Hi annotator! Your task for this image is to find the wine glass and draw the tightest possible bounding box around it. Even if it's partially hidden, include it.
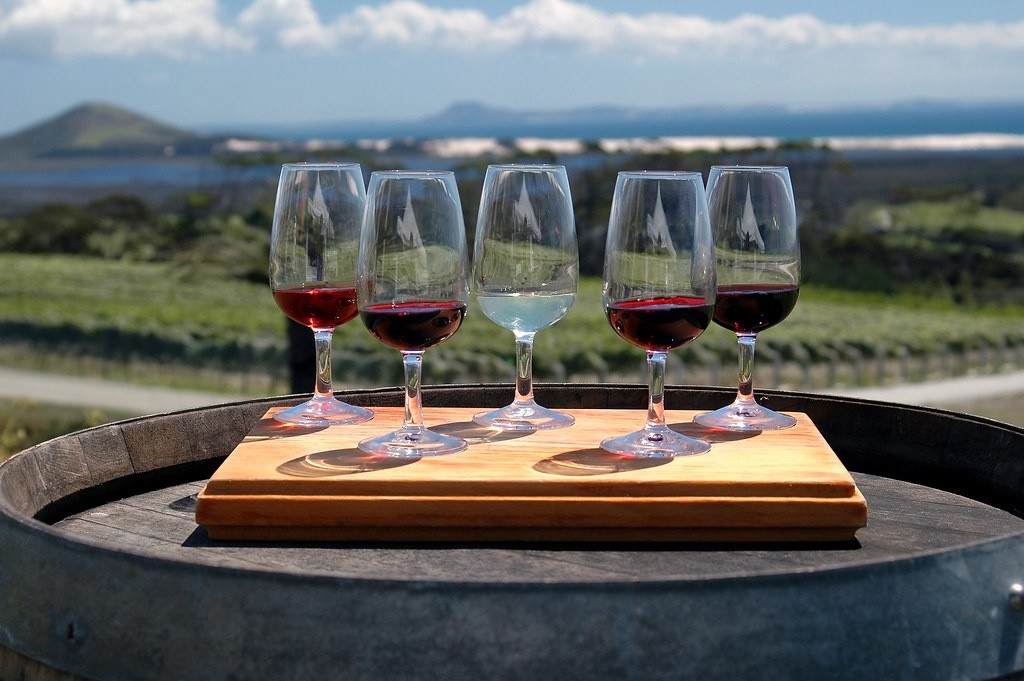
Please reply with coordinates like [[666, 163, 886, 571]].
[[357, 170, 472, 458], [690, 166, 802, 433], [268, 163, 376, 427], [599, 171, 717, 461], [472, 163, 579, 431]]
